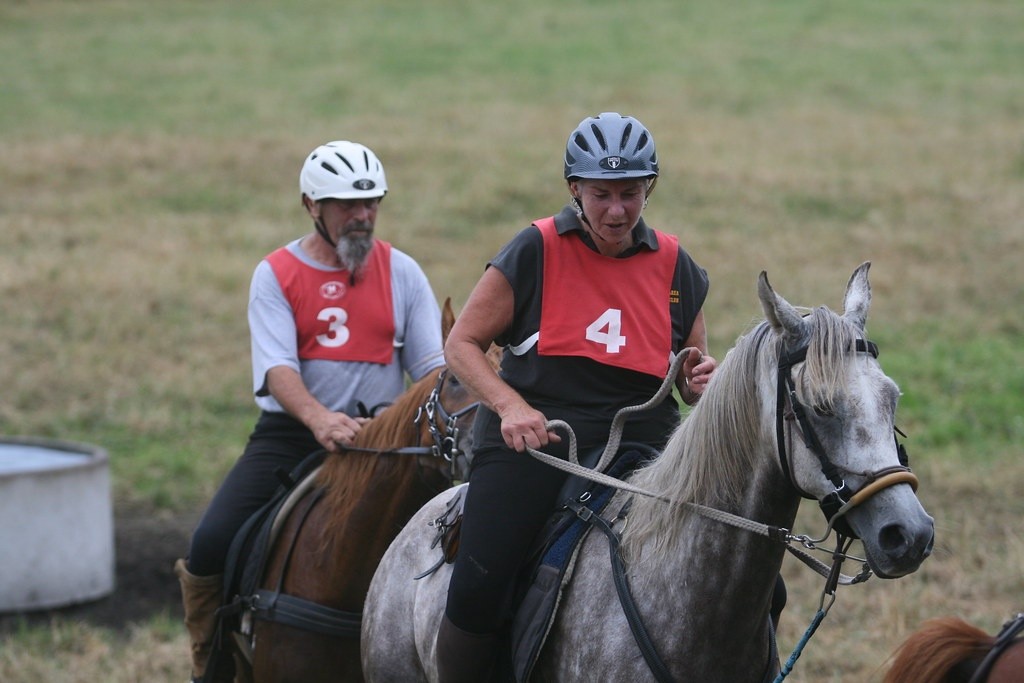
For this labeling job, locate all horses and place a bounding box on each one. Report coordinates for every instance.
[875,618,1024,683]
[228,295,503,682]
[362,261,936,683]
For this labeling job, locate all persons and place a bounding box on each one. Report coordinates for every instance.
[175,139,448,683]
[436,113,718,683]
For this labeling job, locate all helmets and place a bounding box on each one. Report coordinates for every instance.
[563,112,659,181]
[299,140,389,207]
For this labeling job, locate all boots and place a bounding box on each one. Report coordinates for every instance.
[173,559,237,683]
[435,611,496,683]
